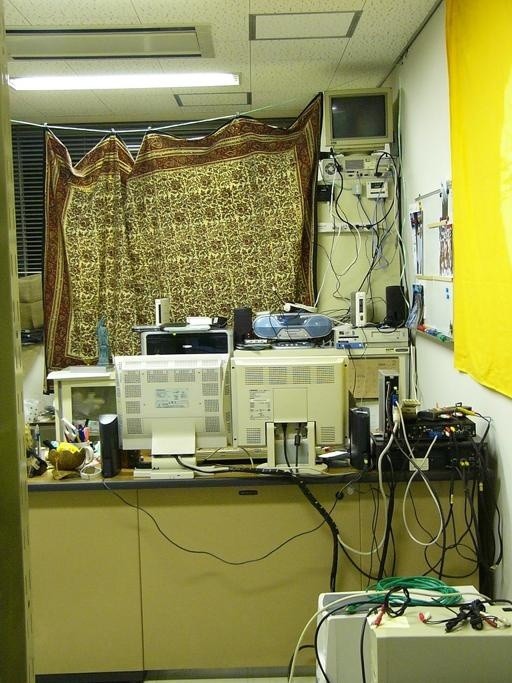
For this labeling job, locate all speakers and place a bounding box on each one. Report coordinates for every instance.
[234,306,252,346]
[99,415,116,479]
[351,405,373,471]
[385,286,404,325]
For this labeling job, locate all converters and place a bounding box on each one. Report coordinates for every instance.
[352,183,362,195]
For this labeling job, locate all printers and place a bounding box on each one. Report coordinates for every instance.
[141,317,233,355]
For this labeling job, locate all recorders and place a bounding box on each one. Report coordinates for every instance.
[253,311,333,341]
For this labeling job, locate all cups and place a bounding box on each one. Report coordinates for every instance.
[70,442,94,465]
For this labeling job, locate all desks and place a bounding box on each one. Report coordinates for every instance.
[25,445,496,677]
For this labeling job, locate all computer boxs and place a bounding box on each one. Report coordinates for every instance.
[316,584,490,683]
[368,605,511,683]
[318,157,396,180]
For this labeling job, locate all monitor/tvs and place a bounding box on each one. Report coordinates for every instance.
[232,350,349,474]
[323,87,394,151]
[114,354,231,479]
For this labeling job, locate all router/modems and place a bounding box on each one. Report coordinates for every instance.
[378,369,399,434]
[350,292,367,328]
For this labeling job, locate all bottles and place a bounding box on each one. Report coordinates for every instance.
[97,320,110,366]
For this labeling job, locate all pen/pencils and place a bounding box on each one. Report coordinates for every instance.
[61,416,91,442]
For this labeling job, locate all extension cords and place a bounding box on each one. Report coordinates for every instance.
[316,223,375,233]
